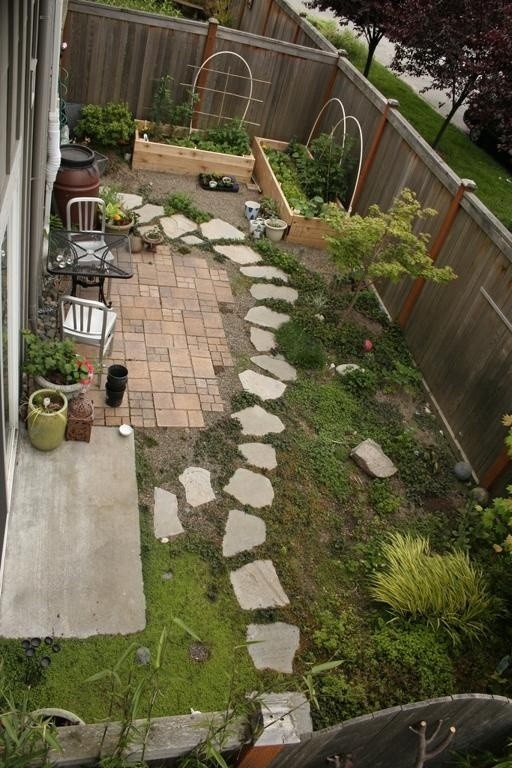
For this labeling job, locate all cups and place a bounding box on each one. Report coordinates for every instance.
[56,249,73,268]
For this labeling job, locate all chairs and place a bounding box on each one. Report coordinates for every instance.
[66,197,114,275]
[59,294,117,390]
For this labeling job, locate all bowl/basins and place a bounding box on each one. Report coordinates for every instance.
[118,424,133,437]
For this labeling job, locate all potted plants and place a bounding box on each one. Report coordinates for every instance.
[97,183,134,235]
[264,216,287,241]
[250,216,265,239]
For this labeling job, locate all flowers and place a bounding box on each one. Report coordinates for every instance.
[18,326,92,384]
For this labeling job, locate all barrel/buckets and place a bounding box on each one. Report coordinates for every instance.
[54,143,100,227]
[104,364,129,408]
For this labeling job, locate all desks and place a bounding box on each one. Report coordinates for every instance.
[46,230,134,308]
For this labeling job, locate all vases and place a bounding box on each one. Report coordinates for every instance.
[245,200,260,220]
[33,368,92,400]
